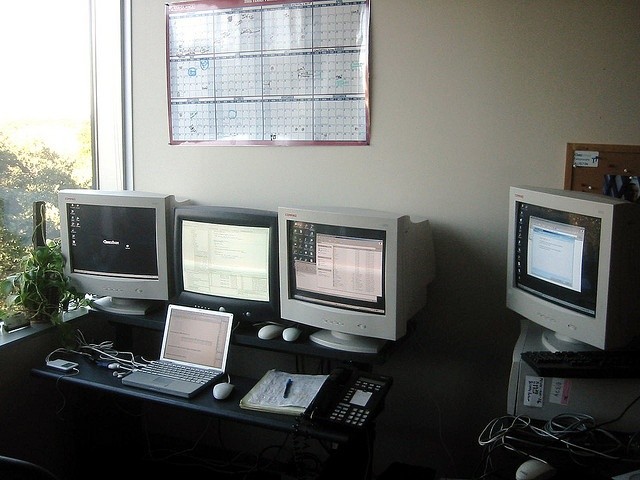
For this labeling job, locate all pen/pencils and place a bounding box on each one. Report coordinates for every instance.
[283,379,291,398]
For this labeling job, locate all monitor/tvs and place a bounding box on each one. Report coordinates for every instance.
[278,205,436,354]
[58,189,191,316]
[173,206,279,322]
[506,185,640,352]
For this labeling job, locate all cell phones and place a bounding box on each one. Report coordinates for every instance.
[46,359,79,373]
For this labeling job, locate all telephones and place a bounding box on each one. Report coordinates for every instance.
[292,366,394,469]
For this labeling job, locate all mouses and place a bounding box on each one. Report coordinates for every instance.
[515,459,557,480]
[258,325,285,340]
[283,328,302,342]
[213,382,235,400]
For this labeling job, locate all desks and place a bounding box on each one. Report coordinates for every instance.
[498,416,640,480]
[29,299,413,480]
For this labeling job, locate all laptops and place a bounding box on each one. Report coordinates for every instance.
[121,304,234,399]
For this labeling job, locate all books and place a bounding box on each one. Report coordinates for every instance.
[239,370,330,415]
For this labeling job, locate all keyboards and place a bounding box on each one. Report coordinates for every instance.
[521,350,640,378]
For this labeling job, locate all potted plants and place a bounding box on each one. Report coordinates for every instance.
[1,241,93,338]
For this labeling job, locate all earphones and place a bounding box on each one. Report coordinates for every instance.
[112,371,124,376]
[117,375,123,378]
